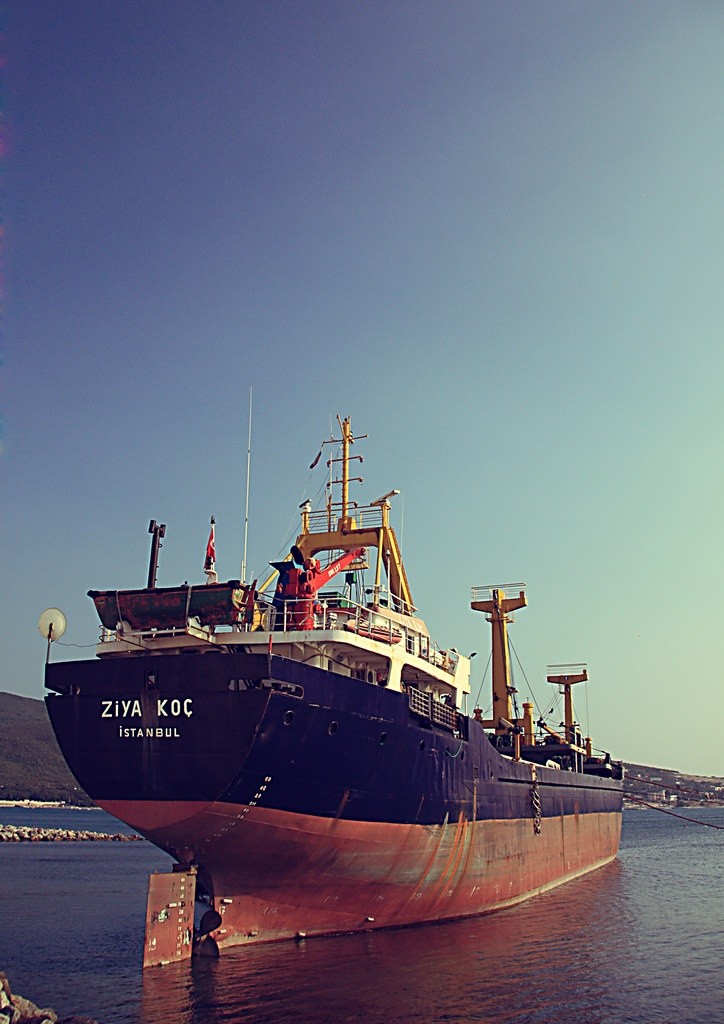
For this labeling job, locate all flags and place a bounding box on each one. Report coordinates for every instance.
[204,523,218,575]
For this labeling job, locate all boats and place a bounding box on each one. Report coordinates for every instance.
[40,406,628,968]
